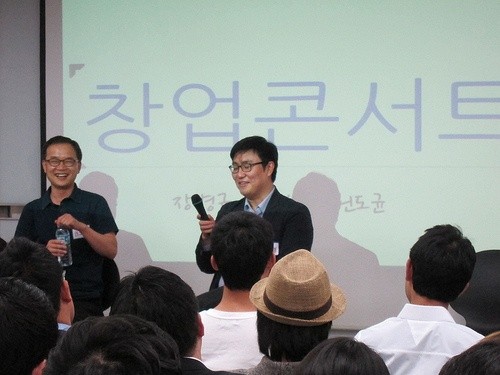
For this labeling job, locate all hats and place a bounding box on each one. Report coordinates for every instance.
[249,249,347,326]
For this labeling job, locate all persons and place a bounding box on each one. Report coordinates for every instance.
[0,276,500,375]
[195,134,314,312]
[12,135,120,328]
[0,211,499,375]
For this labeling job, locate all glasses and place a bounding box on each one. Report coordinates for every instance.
[45,158,79,167]
[229,162,264,174]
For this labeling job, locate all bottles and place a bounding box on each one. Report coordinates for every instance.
[56,227,72,266]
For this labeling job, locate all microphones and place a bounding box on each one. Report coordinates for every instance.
[191,194,210,221]
